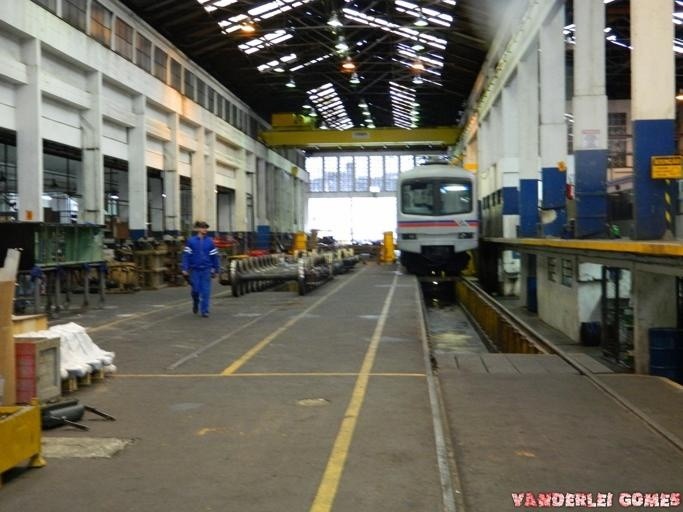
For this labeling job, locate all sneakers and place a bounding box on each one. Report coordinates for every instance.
[192,301,209,318]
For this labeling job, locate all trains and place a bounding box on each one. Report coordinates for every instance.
[396,157,480,273]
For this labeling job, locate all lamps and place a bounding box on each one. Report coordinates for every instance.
[284,79,296,88]
[326,11,361,85]
[411,76,424,85]
[413,7,428,27]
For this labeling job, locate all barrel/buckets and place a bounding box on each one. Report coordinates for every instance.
[648,327,683,385]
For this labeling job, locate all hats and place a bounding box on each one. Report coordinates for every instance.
[194,221,209,229]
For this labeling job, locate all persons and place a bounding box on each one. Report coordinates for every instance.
[181,221,221,317]
